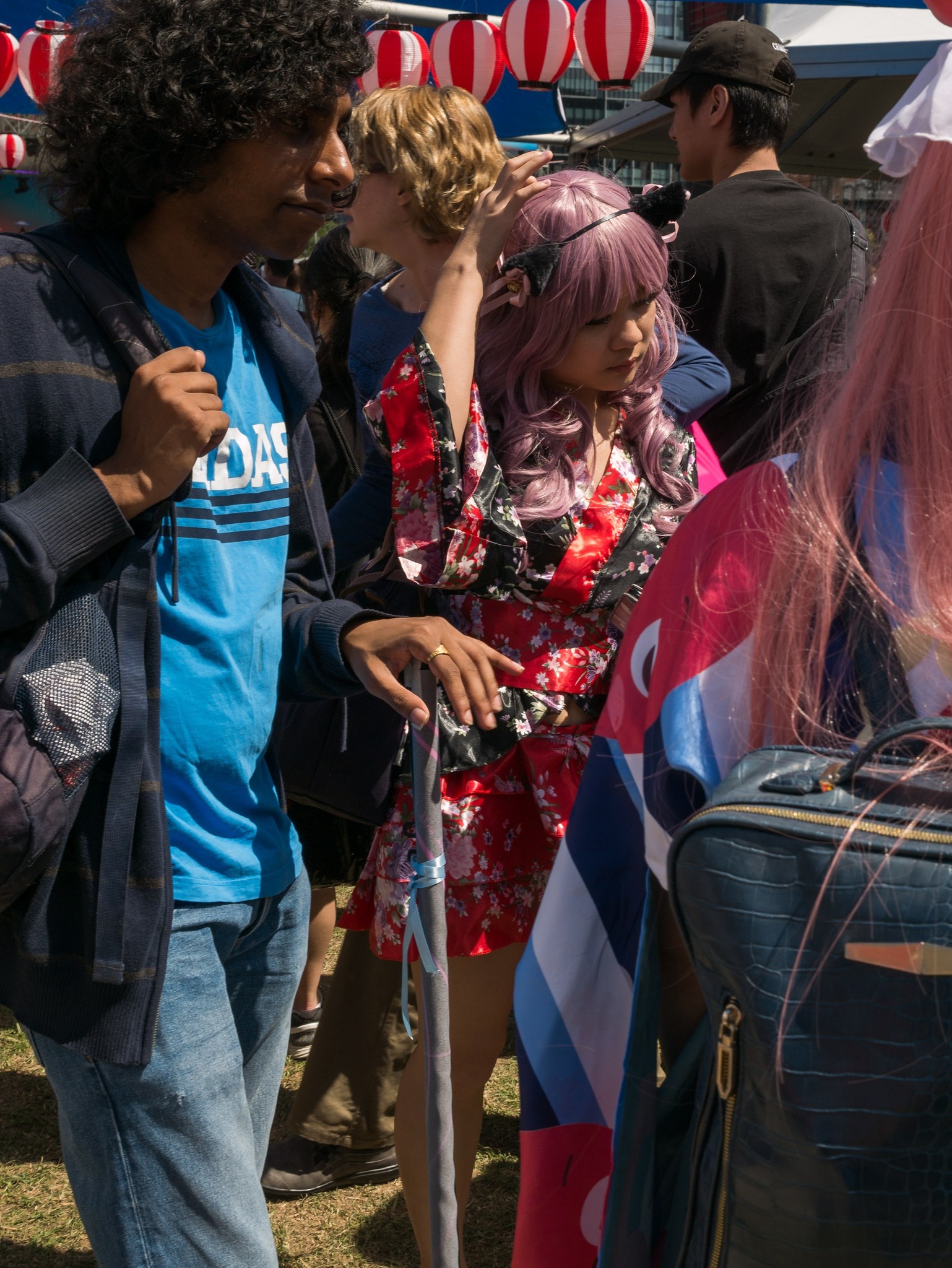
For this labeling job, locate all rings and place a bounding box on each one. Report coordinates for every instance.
[427,643,451,662]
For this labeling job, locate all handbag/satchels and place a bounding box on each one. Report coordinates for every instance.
[1,580,120,908]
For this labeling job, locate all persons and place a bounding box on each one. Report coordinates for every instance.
[366,171,695,1267]
[264,83,731,1268]
[262,223,404,1060]
[0,0,525,1268]
[640,18,874,477]
[509,42,952,1266]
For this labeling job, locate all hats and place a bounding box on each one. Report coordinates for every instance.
[639,21,796,107]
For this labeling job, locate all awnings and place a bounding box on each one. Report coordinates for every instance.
[566,0,952,179]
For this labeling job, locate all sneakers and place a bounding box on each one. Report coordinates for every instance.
[285,1003,321,1061]
[260,1136,399,1194]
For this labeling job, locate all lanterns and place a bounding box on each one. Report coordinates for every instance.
[0,0,84,169]
[355,0,654,106]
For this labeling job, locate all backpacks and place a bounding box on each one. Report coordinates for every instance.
[667,463,952,1268]
